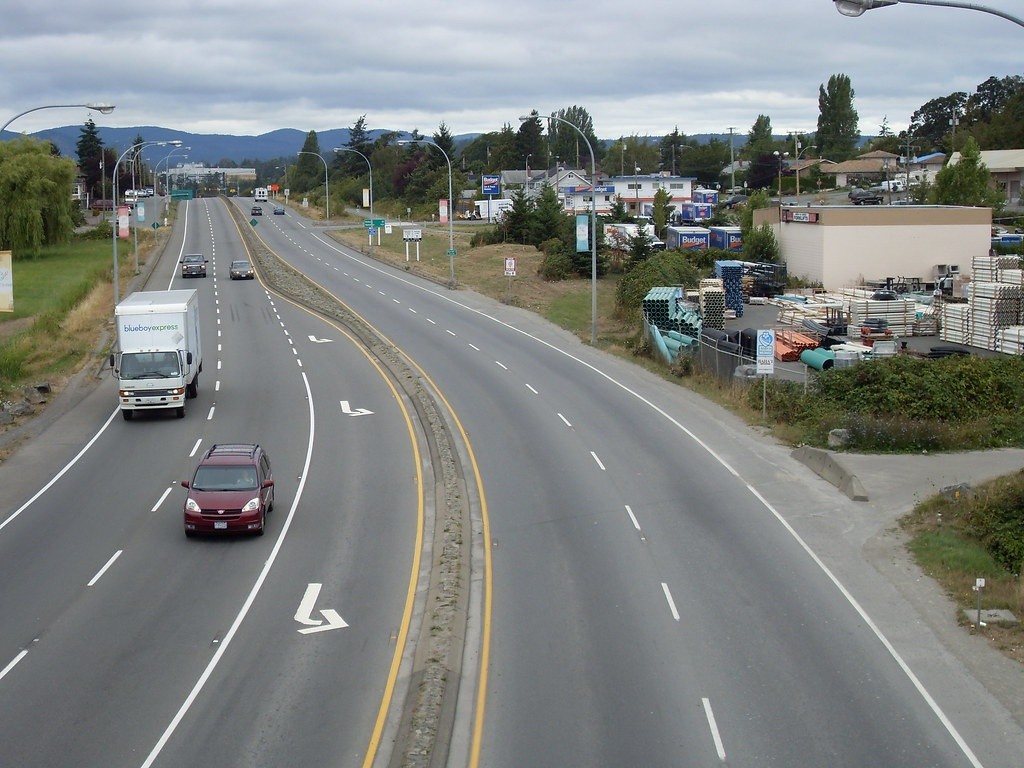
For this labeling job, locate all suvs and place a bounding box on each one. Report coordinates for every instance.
[181,445,273,540]
[180,254,208,278]
[89,200,114,211]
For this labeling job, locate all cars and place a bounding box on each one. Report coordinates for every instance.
[251,207,263,216]
[723,179,926,210]
[273,206,285,216]
[229,261,254,281]
[116,188,153,223]
[521,114,599,348]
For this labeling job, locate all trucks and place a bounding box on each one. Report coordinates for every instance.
[110,290,203,421]
[254,188,268,203]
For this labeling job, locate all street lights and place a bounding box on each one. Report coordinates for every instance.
[166,146,191,216]
[398,140,454,278]
[132,140,184,275]
[773,151,790,204]
[111,139,167,306]
[152,154,188,242]
[796,146,817,205]
[296,149,374,247]
[276,165,295,204]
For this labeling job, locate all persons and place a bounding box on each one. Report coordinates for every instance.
[237,470,253,485]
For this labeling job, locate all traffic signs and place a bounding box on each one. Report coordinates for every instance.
[365,220,386,227]
